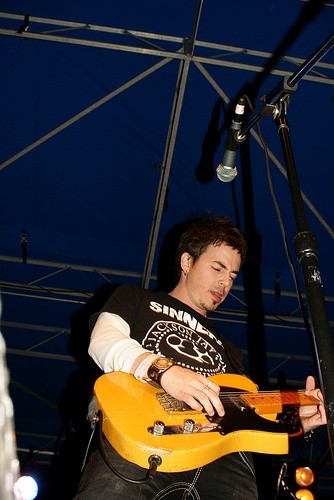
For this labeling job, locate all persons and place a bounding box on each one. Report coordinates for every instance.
[76,216,328,500]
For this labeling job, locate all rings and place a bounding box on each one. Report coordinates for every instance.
[203,386,210,394]
[206,380,213,388]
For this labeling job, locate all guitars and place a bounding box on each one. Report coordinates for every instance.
[93,372,319,472]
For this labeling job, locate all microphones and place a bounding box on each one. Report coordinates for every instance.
[217,98,245,182]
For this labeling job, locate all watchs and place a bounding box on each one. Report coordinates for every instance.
[149,356,175,383]
